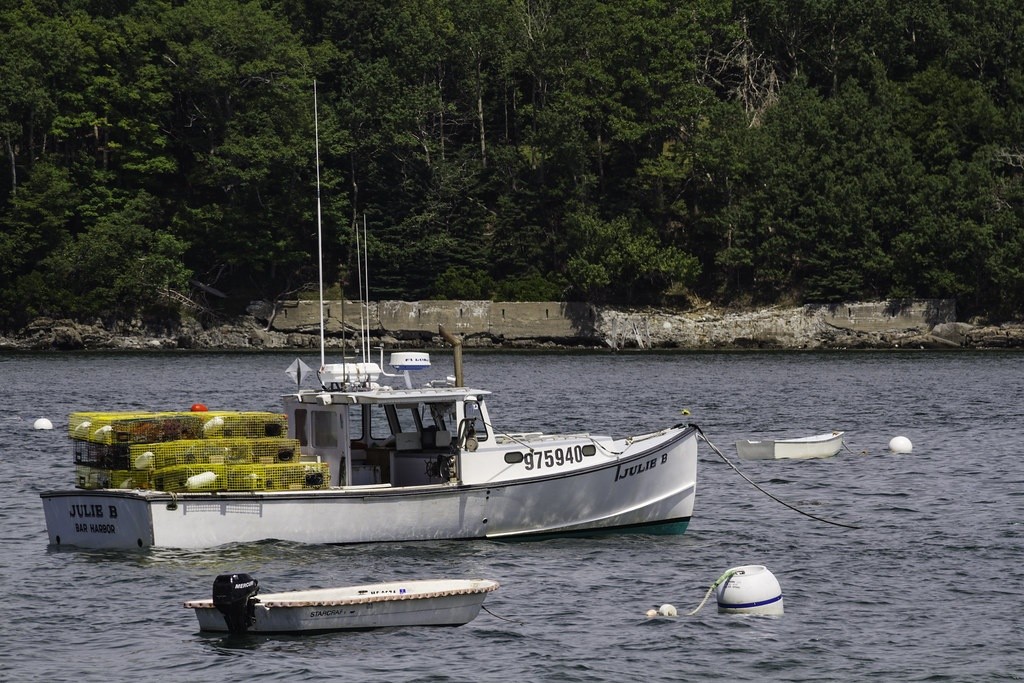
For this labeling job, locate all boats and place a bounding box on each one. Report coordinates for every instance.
[735,430,845,461]
[184,573,500,626]
[41,77,698,551]
